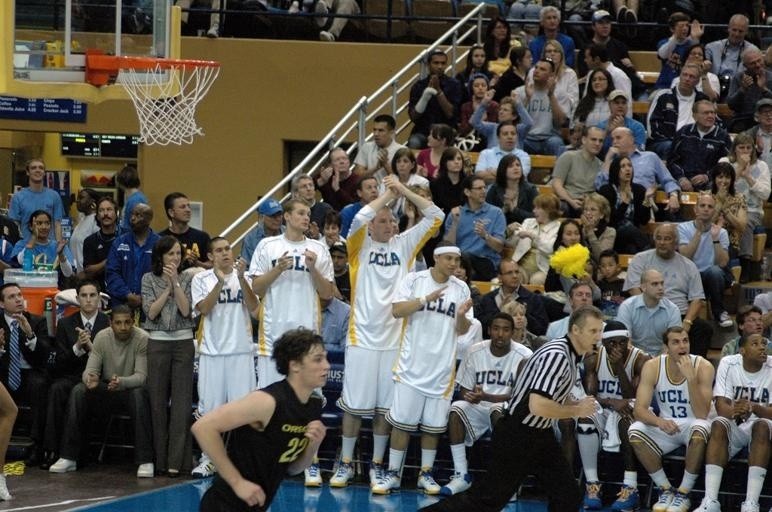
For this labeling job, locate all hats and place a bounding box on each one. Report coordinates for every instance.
[607,88,630,102]
[755,98,772,111]
[592,8,612,23]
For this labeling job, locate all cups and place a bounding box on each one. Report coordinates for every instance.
[35,263,52,277]
[45,297,65,337]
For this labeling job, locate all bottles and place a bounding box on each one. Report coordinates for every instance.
[22,244,35,271]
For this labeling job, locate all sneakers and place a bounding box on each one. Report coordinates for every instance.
[372,470,403,494]
[0,473,15,501]
[192,457,216,478]
[652,489,673,511]
[49,458,77,473]
[330,458,358,488]
[416,466,441,496]
[440,470,474,496]
[611,484,643,512]
[583,479,601,509]
[739,501,762,512]
[666,493,692,512]
[368,461,386,487]
[303,458,322,488]
[136,463,154,477]
[693,498,721,512]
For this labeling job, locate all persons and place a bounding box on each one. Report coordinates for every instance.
[1,0,772,512]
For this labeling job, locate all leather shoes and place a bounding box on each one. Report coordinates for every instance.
[168,467,181,478]
